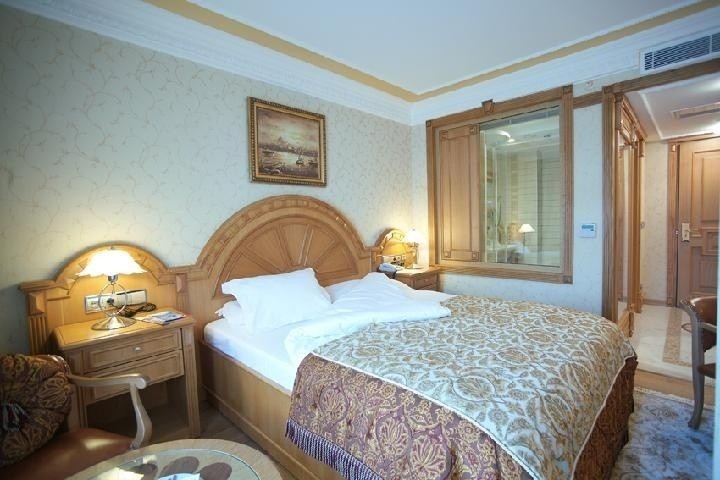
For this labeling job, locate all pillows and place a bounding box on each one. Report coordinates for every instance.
[213,266,349,331]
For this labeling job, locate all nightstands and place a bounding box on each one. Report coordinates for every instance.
[394,263,444,293]
[51,309,199,439]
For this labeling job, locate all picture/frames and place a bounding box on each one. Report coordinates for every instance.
[245,95,328,188]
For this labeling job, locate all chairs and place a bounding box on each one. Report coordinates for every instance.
[678,294,718,432]
[0,352,155,479]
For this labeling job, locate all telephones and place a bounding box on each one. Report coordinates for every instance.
[379,263,403,273]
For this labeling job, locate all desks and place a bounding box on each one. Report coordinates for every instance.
[64,437,284,480]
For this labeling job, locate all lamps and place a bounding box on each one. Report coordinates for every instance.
[402,227,422,269]
[76,244,150,331]
[517,220,536,253]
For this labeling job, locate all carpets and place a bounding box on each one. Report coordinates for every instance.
[610,383,716,479]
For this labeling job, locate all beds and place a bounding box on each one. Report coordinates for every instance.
[196,193,640,480]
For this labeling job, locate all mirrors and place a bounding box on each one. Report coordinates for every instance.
[424,79,577,285]
